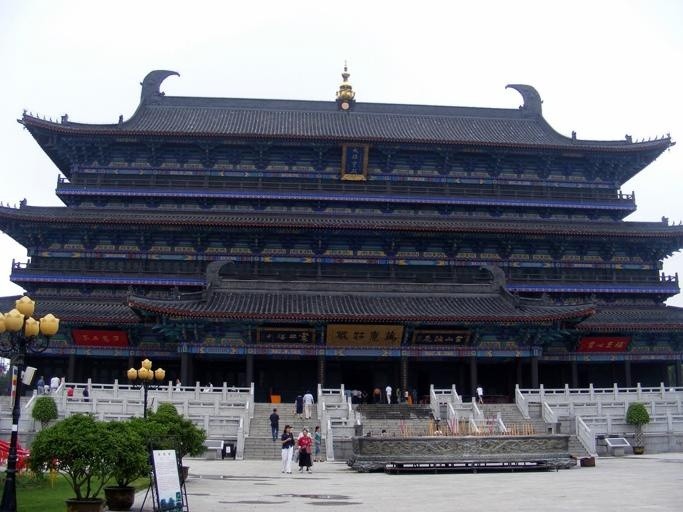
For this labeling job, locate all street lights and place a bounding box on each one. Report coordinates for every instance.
[126,359,166,420]
[1,296,59,511]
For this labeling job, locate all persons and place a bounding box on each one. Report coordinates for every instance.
[476,383,485,405]
[344,382,418,406]
[433,426,443,435]
[50,374,60,392]
[380,429,386,436]
[82,385,90,402]
[66,384,74,401]
[37,375,45,396]
[268,389,323,475]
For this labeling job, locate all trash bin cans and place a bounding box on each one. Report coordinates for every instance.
[221,443,236,460]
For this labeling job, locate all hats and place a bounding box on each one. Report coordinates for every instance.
[285,425,292,428]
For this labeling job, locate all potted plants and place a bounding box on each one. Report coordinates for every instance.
[626,403,650,454]
[97,418,152,512]
[132,403,208,487]
[24,414,125,512]
